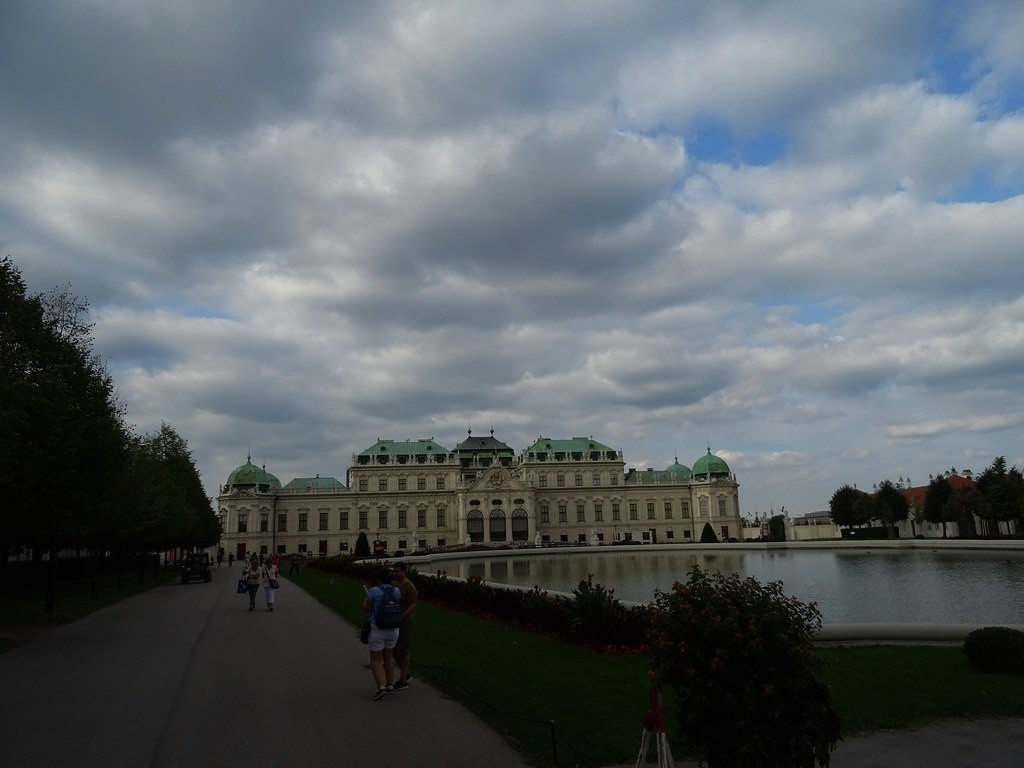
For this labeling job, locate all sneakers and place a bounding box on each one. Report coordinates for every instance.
[371,688,394,700]
[406,674,412,683]
[394,680,408,690]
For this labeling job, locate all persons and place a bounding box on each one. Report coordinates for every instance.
[229,552,234,566]
[217,553,222,567]
[510,540,528,548]
[363,561,418,701]
[261,558,278,612]
[242,560,262,611]
[424,544,449,552]
[301,549,312,556]
[578,539,580,546]
[350,547,352,555]
[244,551,302,578]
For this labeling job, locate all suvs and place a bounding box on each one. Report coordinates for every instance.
[180,553,212,584]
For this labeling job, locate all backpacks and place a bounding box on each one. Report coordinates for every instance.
[372,585,400,630]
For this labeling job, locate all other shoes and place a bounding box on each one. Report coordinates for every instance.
[297,574,299,576]
[289,574,292,577]
[248,606,254,611]
[266,607,273,611]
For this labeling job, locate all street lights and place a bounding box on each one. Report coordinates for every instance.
[272,494,277,554]
[688,484,695,543]
[376,533,379,540]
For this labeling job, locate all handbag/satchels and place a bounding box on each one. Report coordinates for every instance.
[237,580,247,593]
[270,578,280,589]
